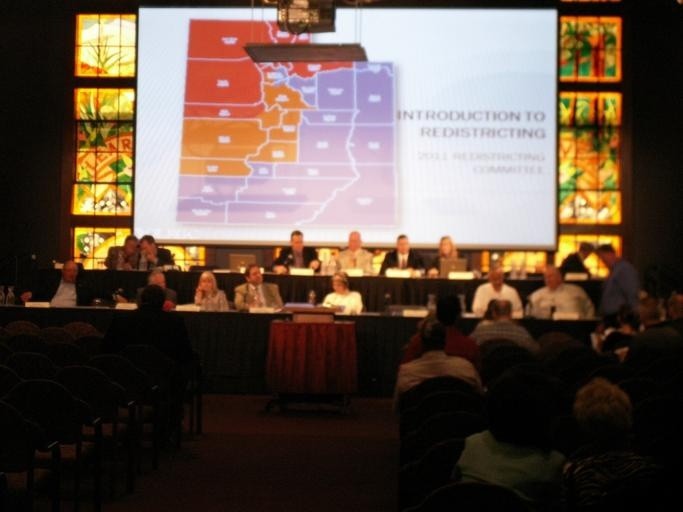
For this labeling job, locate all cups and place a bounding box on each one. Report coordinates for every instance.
[96,261,103,269]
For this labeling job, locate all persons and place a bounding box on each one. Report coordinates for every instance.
[20,260,103,306]
[427,236,472,277]
[332,232,374,275]
[389,294,682,512]
[525,266,593,317]
[102,285,193,448]
[193,270,230,312]
[103,235,140,271]
[234,263,284,313]
[595,242,642,331]
[560,242,595,279]
[320,270,362,313]
[140,236,175,272]
[271,231,321,274]
[378,234,424,277]
[472,259,522,311]
[135,273,178,309]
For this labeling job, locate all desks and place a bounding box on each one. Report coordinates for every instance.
[0,263,606,400]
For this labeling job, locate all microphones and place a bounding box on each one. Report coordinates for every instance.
[549,306,557,321]
[385,293,391,306]
[112,288,123,296]
[282,254,293,265]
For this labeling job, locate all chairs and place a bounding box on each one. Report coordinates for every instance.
[0,319,203,512]
[393,322,683,512]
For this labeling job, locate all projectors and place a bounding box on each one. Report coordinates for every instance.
[276,0,336,33]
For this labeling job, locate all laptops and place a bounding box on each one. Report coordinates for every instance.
[439,257,468,279]
[228,253,257,274]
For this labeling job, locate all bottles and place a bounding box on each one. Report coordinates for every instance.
[524,300,535,317]
[508,258,527,280]
[138,251,146,270]
[5,286,14,304]
[305,289,315,305]
[239,262,245,274]
[383,295,392,316]
[328,253,340,274]
[115,249,124,269]
